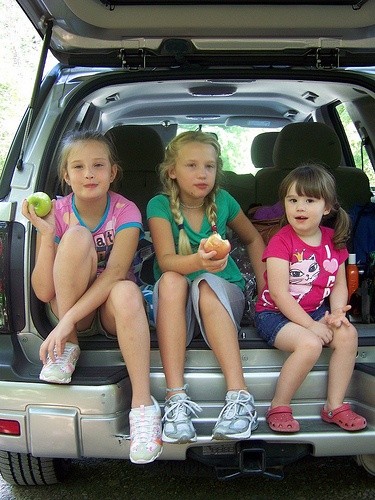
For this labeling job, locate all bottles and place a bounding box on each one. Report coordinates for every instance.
[345,253,359,304]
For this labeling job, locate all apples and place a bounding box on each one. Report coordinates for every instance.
[204,234,231,259]
[26,191,51,217]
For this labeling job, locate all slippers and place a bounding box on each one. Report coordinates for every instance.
[321,403,366,430]
[266,406,300,432]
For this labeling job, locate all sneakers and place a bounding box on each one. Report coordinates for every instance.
[212,390,259,439]
[129,395,163,464]
[39,341,80,383]
[162,393,202,443]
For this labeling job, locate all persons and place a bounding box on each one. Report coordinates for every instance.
[255,166,367,432]
[22,130,164,464]
[147,131,267,444]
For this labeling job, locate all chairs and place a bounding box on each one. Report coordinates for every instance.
[250,133,280,169]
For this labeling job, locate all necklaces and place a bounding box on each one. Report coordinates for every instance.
[180,201,205,208]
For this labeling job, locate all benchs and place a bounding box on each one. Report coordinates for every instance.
[101,122,371,233]
